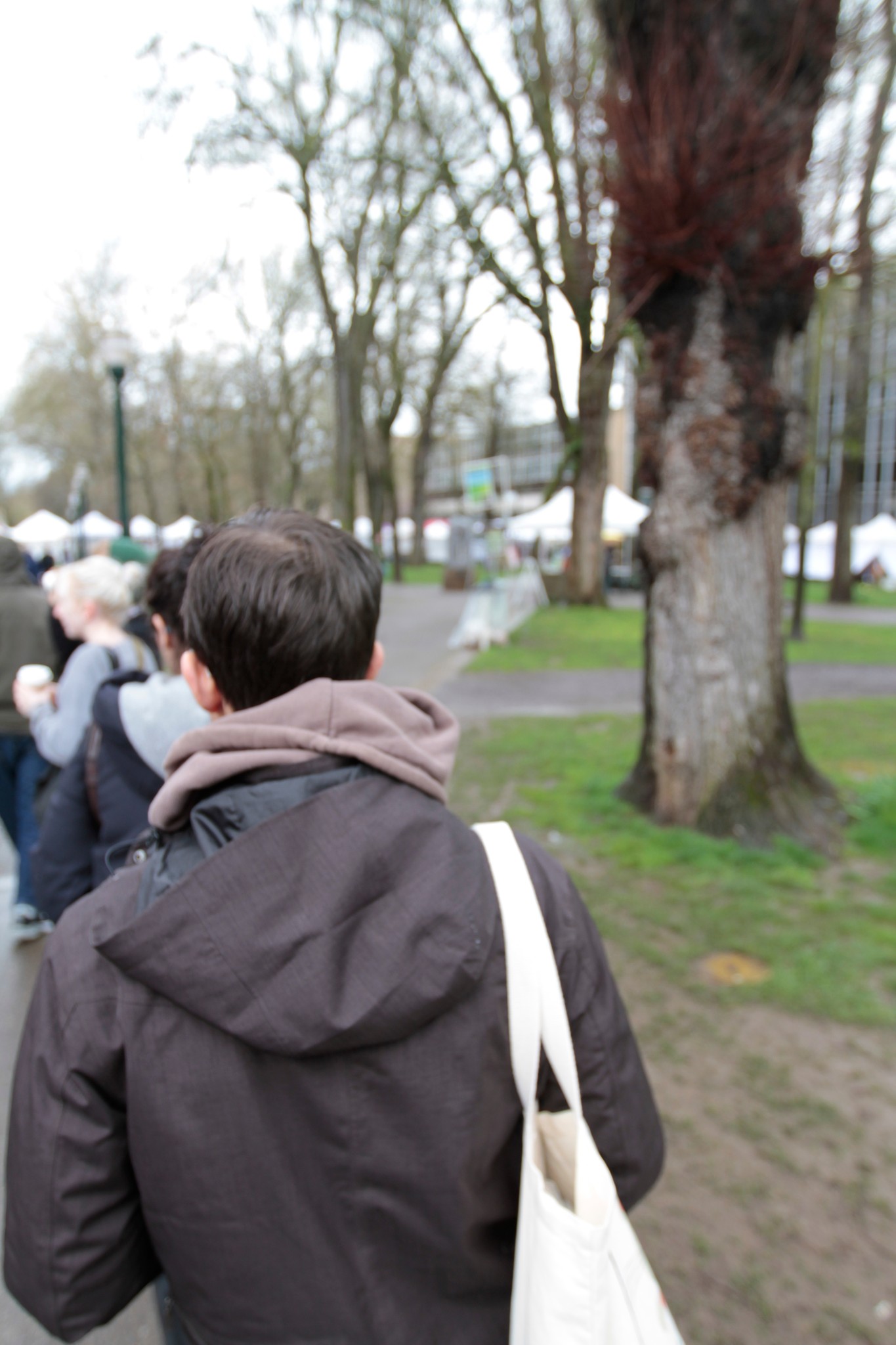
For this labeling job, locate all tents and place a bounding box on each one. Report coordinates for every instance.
[0,468,896,602]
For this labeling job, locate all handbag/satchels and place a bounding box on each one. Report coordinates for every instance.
[470,815,686,1345]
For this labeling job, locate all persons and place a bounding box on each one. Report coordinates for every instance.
[1,506,665,1345]
[29,531,231,934]
[0,526,160,943]
